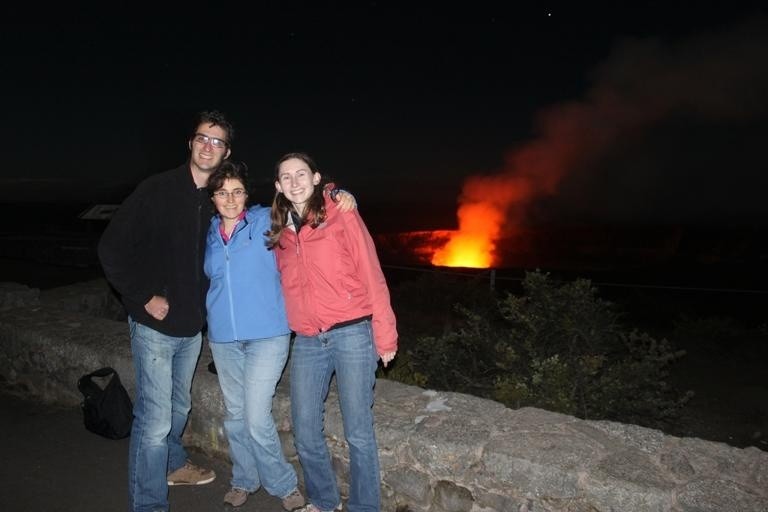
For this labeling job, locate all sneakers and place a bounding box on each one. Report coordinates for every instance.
[164,459,216,486]
[280,488,344,512]
[225,484,261,507]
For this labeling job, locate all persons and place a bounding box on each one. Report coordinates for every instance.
[262,153,399,512]
[203,161,359,511]
[98,111,234,512]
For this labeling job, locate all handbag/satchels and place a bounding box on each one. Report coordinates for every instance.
[77,368,133,439]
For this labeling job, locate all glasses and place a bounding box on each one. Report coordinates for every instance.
[192,133,230,149]
[213,189,247,199]
